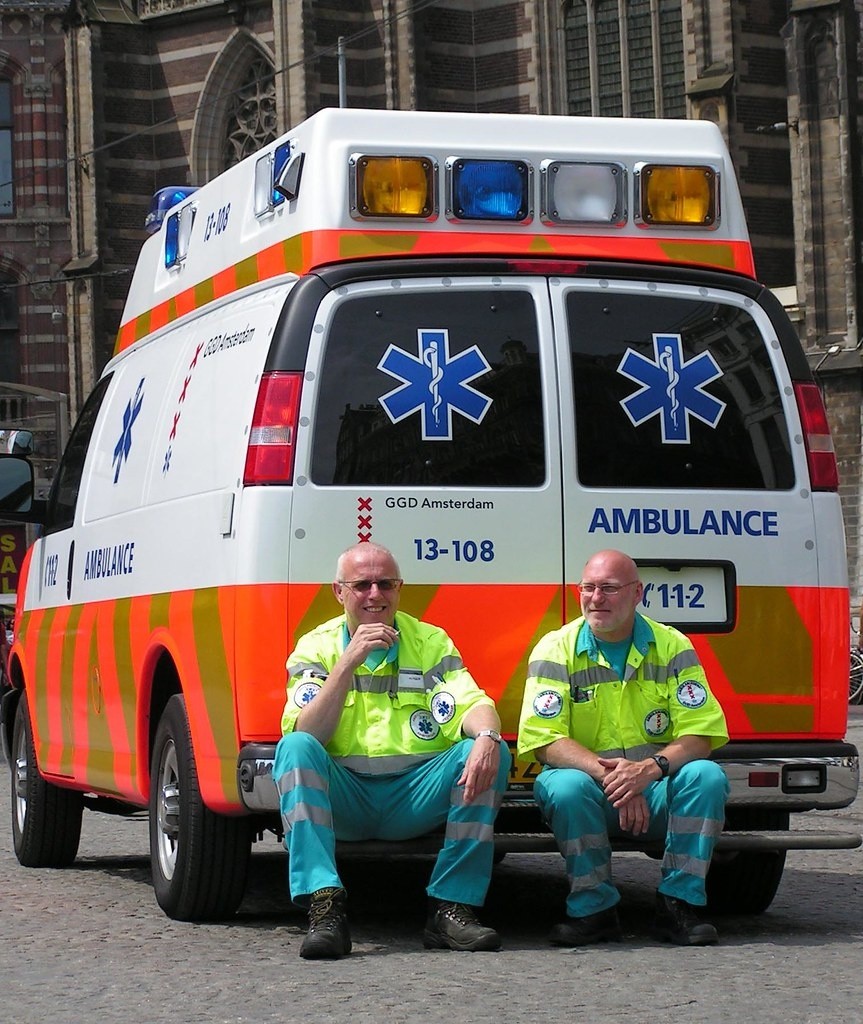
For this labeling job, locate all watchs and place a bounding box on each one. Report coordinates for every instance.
[477,730,501,744]
[650,756,669,778]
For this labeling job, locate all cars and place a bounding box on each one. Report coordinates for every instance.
[0,592,20,643]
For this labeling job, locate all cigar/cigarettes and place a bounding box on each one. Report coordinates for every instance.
[394,630,401,635]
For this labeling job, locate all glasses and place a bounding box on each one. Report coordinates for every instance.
[576,580,638,595]
[339,579,402,592]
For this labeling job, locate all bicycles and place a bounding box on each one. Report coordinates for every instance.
[845,647,863,703]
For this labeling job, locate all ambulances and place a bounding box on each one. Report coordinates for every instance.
[1,100,861,922]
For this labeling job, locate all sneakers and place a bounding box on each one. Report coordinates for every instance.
[300,887,352,958]
[549,906,620,945]
[423,896,501,951]
[653,887,718,946]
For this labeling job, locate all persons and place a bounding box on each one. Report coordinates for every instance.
[0,612,14,686]
[274,542,512,956]
[517,549,731,944]
